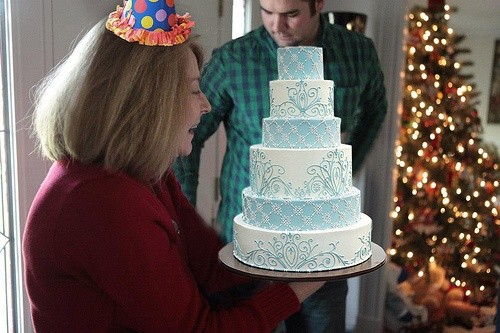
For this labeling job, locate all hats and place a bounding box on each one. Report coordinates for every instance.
[106,0,195,44]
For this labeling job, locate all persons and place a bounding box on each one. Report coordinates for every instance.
[171,0,388,333]
[20,14,327,333]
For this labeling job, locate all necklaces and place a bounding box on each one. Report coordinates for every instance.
[149,182,180,235]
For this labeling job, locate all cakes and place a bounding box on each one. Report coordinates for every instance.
[232,47,374,273]
